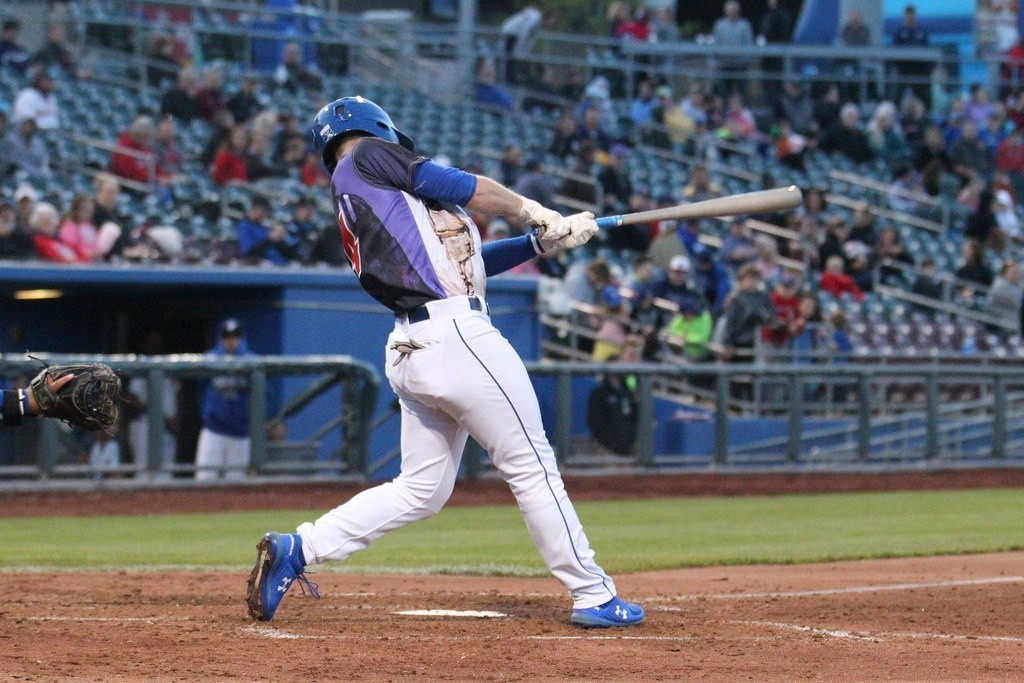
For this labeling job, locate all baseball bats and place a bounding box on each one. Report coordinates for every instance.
[529,184,804,239]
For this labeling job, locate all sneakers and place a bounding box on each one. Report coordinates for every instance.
[570,596,645,626]
[245,532,320,622]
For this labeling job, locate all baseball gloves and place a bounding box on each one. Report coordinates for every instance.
[32,360,124,434]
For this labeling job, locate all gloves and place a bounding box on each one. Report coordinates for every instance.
[508,196,571,240]
[542,211,599,256]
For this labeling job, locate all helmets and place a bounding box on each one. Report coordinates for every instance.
[223,319,240,334]
[311,96,413,177]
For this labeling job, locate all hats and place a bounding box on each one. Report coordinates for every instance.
[669,255,690,272]
[610,144,629,160]
[15,186,35,203]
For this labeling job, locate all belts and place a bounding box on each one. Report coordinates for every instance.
[407,296,481,325]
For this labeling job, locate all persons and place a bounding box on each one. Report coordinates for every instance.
[275,41,322,92]
[587,340,639,454]
[161,60,330,189]
[0,19,26,70]
[149,35,180,87]
[36,22,71,66]
[158,119,181,166]
[503,7,557,90]
[0,354,121,440]
[538,67,555,95]
[1,172,346,267]
[551,0,1024,402]
[469,138,561,278]
[193,317,253,481]
[110,116,182,186]
[472,56,515,108]
[83,372,187,480]
[245,96,645,627]
[13,66,60,130]
